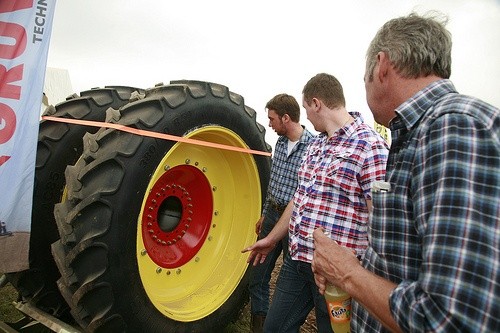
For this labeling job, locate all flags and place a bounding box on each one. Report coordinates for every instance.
[0,0,57,276]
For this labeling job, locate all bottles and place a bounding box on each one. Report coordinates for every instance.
[324,277,353,333]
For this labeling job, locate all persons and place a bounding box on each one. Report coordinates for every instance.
[309,10,500,333]
[240,70,394,333]
[245,93,319,333]
